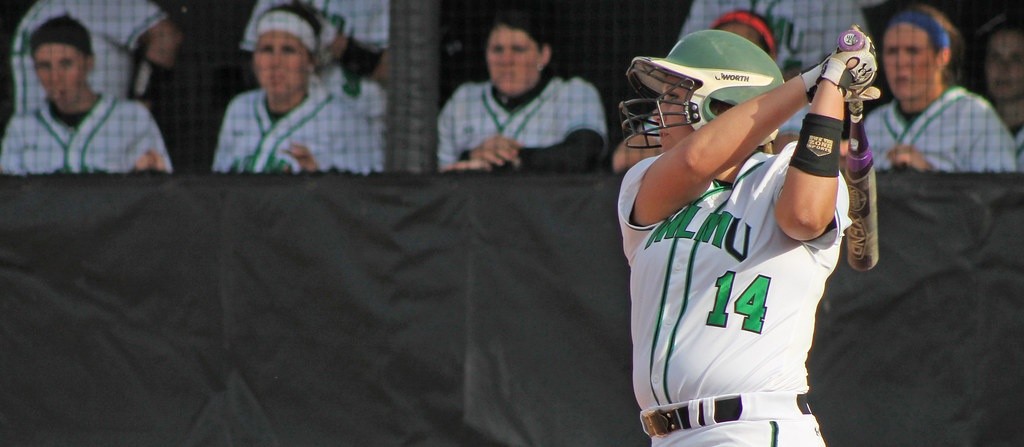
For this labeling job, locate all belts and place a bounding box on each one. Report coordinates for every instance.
[642,393,815,438]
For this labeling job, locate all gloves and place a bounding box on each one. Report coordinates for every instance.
[799,23,881,104]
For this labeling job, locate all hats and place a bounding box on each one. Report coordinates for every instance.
[31,13,92,56]
[256,2,320,52]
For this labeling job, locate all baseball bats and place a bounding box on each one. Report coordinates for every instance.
[837,29,879,270]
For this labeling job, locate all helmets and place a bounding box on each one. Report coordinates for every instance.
[618,30,784,145]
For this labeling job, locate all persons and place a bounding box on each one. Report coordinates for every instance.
[617,24,881,447]
[0,0,182,174]
[436,12,609,171]
[612,1,1023,173]
[211,0,390,176]
